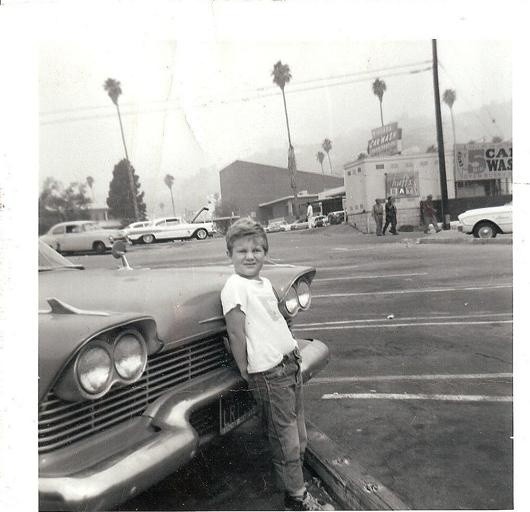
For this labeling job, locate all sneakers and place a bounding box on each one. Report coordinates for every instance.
[285,488,335,510]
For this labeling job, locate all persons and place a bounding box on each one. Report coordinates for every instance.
[221,217,335,510]
[306,201,316,229]
[383,197,399,235]
[374,198,384,237]
[421,194,442,235]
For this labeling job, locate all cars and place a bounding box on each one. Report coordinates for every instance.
[39,240,329,511]
[457,201,514,238]
[266,211,345,233]
[122,217,215,244]
[39,220,132,255]
